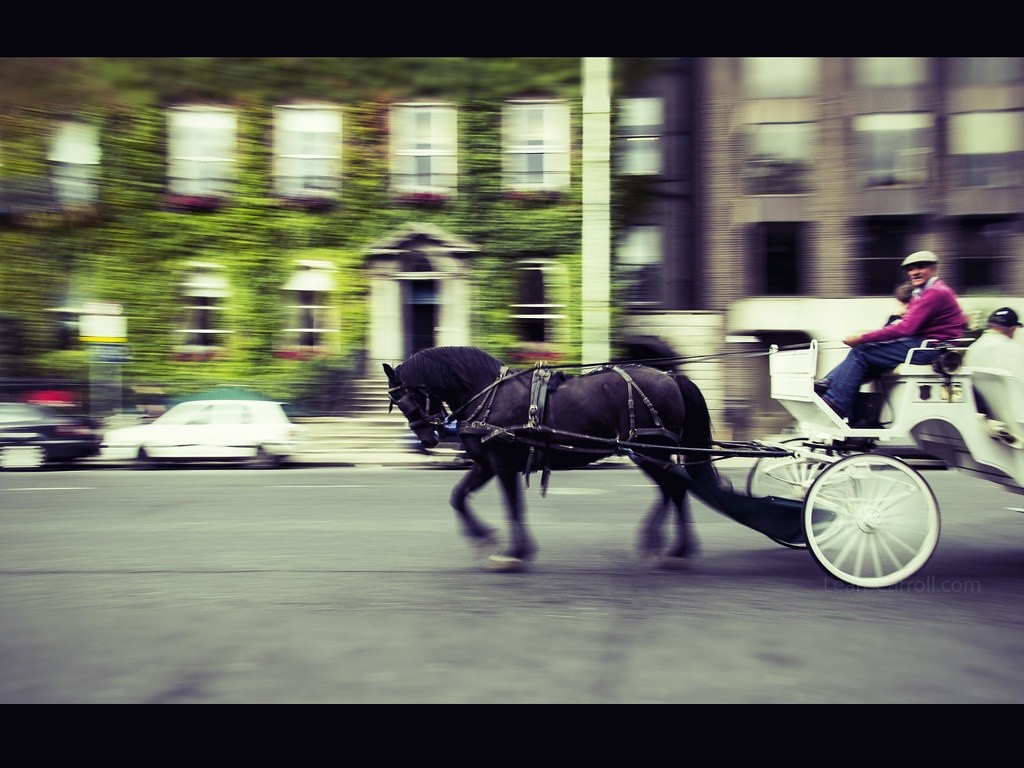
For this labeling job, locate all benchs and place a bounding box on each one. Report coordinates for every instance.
[859,337,979,428]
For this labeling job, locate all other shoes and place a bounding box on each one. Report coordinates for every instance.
[814,381,827,397]
[815,390,850,426]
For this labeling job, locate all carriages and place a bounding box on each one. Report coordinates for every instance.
[381,312,1024,588]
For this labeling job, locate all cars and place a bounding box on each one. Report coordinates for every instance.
[98,401,292,467]
[0,403,103,471]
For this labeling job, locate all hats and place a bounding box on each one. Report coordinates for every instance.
[901,251,938,266]
[988,307,1022,327]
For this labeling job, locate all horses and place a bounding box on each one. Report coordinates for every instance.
[382,346,725,572]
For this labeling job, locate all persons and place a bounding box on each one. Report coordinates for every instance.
[962,307,1024,440]
[814,251,968,420]
